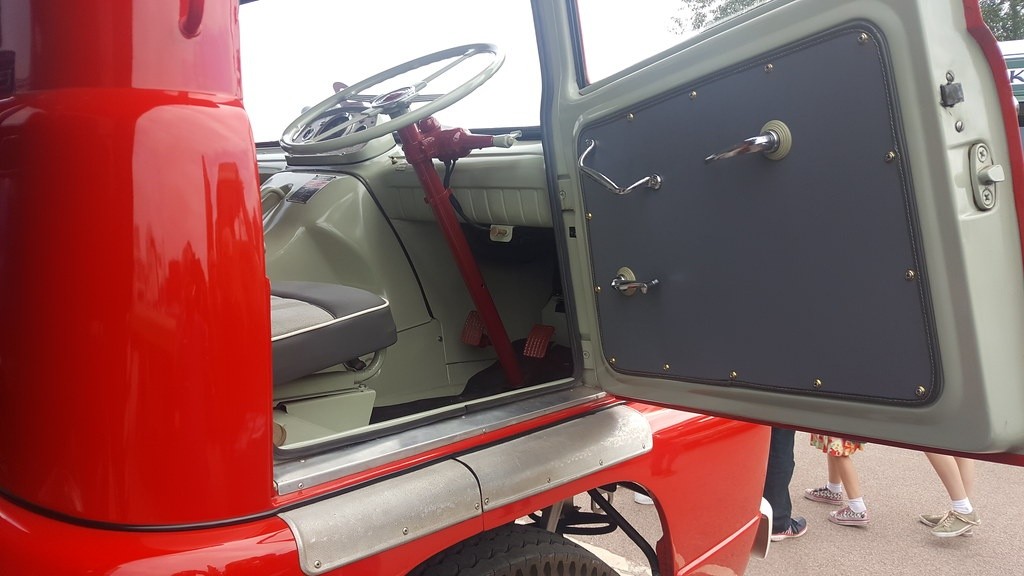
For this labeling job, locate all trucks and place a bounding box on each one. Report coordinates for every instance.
[1,0,1024,576]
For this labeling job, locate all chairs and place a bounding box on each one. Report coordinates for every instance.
[268,280,397,404]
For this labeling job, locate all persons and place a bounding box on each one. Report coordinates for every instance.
[804,433,870,527]
[763,426,807,541]
[919,451,981,538]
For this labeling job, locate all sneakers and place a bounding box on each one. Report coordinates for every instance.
[771,516,808,542]
[803,485,843,505]
[828,506,870,525]
[634,492,654,505]
[918,509,981,538]
